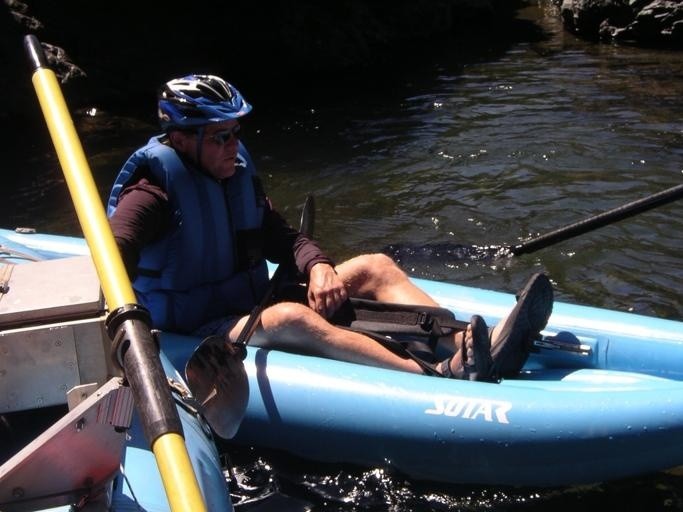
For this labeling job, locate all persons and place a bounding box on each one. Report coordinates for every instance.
[107,75,553,381]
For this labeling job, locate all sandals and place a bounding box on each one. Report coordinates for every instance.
[423,272,553,380]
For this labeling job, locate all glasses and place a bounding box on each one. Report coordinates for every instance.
[214,125,240,146]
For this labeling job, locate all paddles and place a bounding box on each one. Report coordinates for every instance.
[184,187,314,439]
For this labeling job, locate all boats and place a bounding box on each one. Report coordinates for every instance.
[2,230,682,487]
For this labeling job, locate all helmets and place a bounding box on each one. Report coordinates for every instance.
[158,74,253,128]
